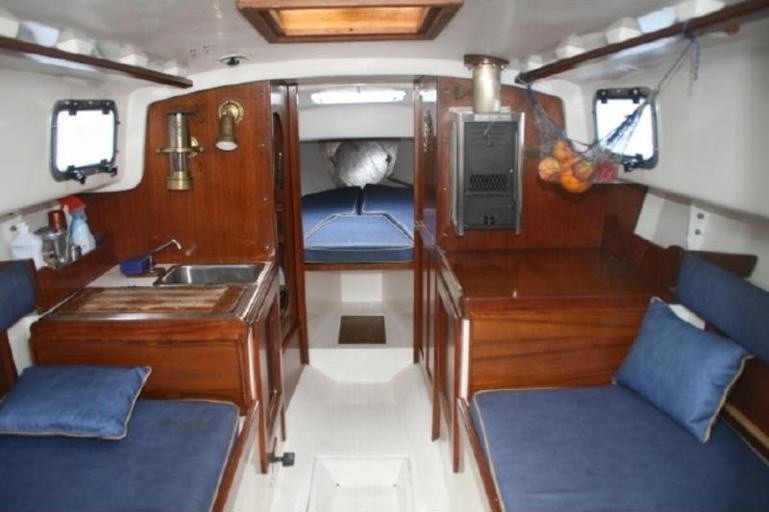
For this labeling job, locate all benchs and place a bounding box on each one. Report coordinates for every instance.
[458,246,768,512]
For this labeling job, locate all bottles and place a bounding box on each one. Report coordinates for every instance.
[34,224,68,268]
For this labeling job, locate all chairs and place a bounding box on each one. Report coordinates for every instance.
[1,259,260,511]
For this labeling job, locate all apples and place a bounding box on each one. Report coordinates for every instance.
[537,139,617,194]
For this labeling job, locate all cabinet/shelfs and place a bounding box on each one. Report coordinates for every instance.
[28,267,304,475]
[74,80,310,413]
[433,249,673,474]
[413,75,649,403]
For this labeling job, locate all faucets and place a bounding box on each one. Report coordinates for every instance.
[146,238,182,270]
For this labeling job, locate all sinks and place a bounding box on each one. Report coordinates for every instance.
[153,261,272,285]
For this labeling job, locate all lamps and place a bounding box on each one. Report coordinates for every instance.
[156,104,204,192]
[215,99,244,152]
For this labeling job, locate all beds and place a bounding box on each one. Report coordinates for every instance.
[299,183,414,350]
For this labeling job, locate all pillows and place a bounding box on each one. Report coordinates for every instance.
[1,363,153,444]
[611,293,755,446]
